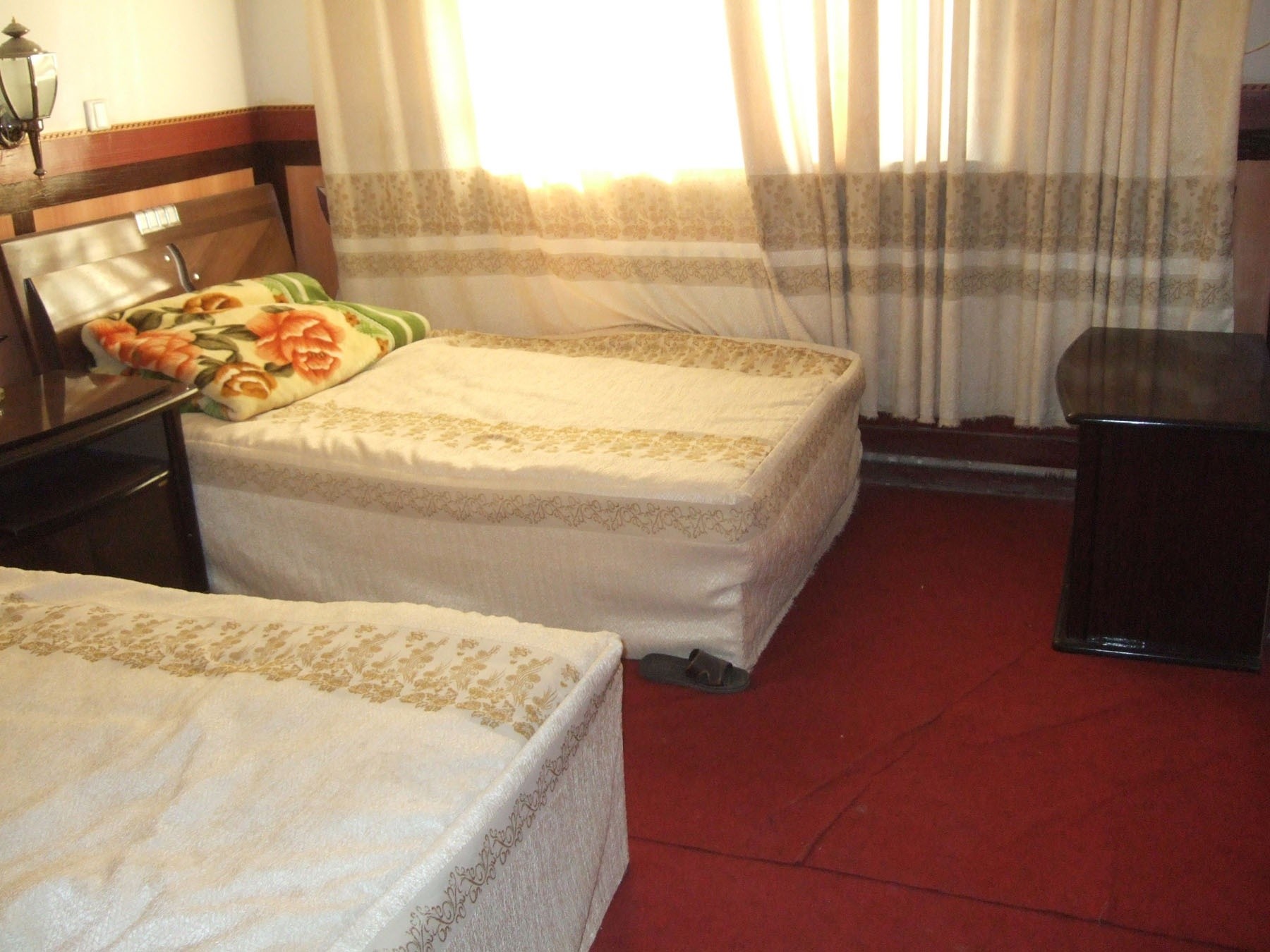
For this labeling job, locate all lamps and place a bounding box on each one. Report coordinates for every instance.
[0,17,60,182]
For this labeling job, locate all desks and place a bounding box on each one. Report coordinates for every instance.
[1047,323,1269,675]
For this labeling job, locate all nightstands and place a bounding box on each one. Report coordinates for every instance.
[0,368,208,593]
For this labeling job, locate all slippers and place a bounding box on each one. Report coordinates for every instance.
[640,648,750,693]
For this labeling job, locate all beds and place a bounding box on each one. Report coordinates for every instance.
[0,566,634,952]
[0,181,867,678]
